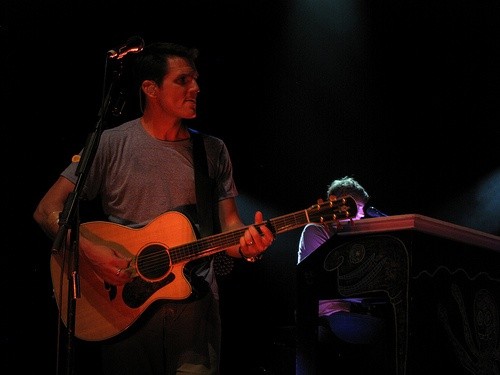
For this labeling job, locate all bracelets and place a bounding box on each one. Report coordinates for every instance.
[238,245,265,264]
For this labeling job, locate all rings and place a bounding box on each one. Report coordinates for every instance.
[116,269,120,276]
[247,241,254,246]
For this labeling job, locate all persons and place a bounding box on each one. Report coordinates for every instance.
[33,42,274,375]
[297,176,383,344]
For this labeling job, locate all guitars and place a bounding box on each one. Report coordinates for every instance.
[49,191,360,342]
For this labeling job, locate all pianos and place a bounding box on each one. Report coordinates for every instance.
[325,214,500,252]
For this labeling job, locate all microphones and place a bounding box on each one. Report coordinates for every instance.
[108,37,144,58]
[367,207,387,217]
